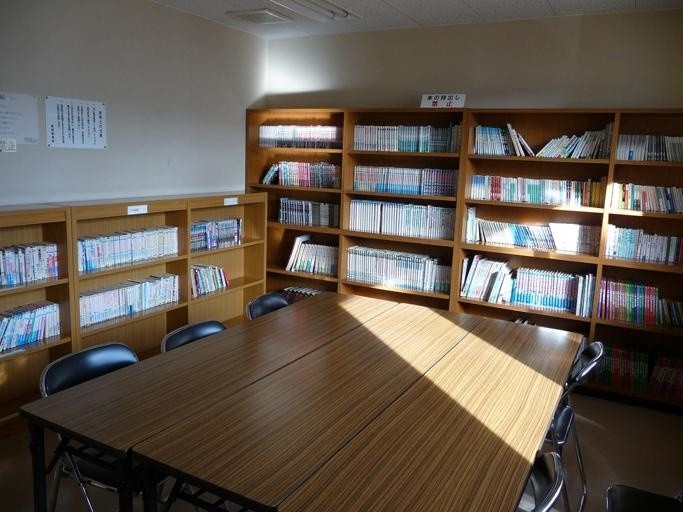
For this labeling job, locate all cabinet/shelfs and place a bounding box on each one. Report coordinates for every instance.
[177,191,267,330]
[0,201,74,434]
[453,106,673,412]
[48,194,189,366]
[245,107,466,314]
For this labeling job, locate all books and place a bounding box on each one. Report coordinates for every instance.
[460,207,601,318]
[282,287,321,304]
[278,198,338,275]
[352,124,462,196]
[0,213,245,355]
[347,199,457,292]
[258,124,341,189]
[469,122,613,208]
[610,132,683,215]
[596,223,682,328]
[591,334,683,407]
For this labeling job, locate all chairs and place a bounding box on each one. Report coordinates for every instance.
[159,295,290,352]
[39,342,195,512]
[514,339,604,512]
[605,483,683,511]
